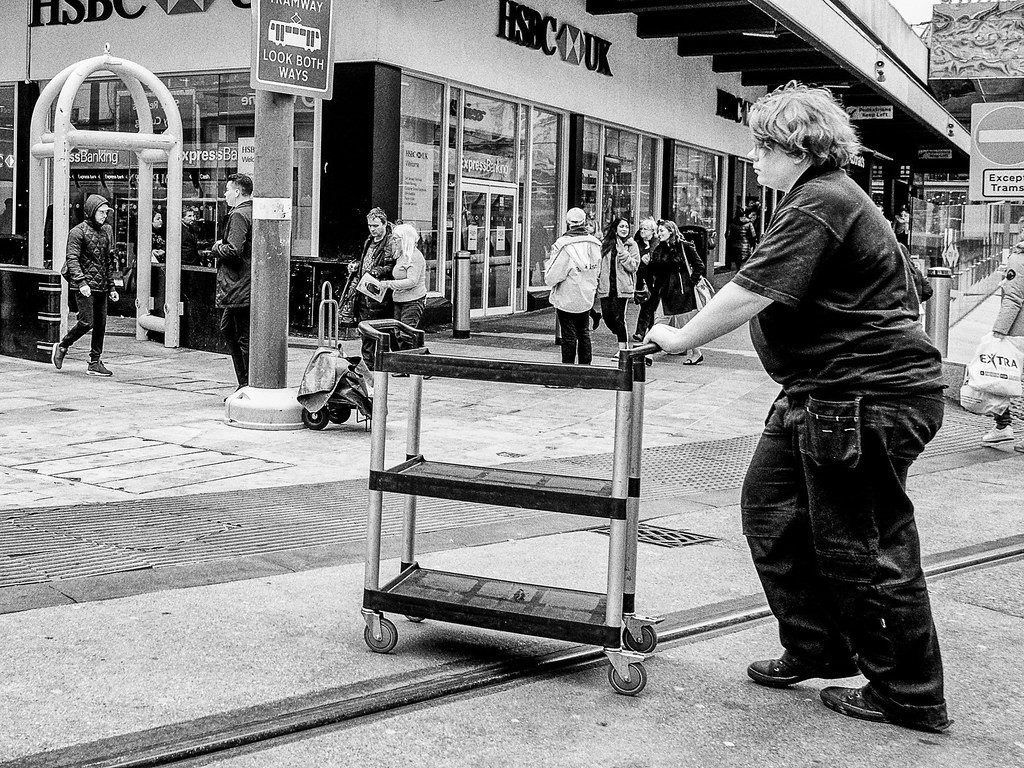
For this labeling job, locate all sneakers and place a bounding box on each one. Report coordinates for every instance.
[86,359,112,376]
[820,686,941,731]
[982,424,1014,442]
[1015,440,1024,452]
[51,342,68,369]
[748,651,861,687]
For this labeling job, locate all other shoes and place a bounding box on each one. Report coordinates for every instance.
[392,370,410,377]
[632,333,643,341]
[223,382,248,403]
[610,351,620,361]
[423,374,431,379]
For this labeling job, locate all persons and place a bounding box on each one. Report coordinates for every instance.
[983,216,1024,452]
[645,79,957,734]
[480,216,550,286]
[545,206,603,390]
[348,204,433,379]
[723,206,757,272]
[876,201,909,248]
[39,193,199,377]
[212,174,253,403]
[582,217,703,365]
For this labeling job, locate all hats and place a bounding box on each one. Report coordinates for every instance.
[567,207,586,226]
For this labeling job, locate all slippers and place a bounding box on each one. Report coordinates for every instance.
[683,353,703,365]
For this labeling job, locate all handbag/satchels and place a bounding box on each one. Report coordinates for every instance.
[635,283,651,305]
[61,259,70,283]
[339,275,360,324]
[694,274,716,311]
[968,333,1022,397]
[960,364,1011,416]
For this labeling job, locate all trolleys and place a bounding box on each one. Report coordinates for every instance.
[360,320,663,694]
[303,281,388,431]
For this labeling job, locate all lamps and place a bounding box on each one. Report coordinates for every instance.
[876,69,886,82]
[948,129,954,137]
[823,82,854,88]
[742,30,780,38]
[874,44,886,70]
[948,114,954,128]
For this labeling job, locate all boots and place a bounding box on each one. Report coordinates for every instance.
[590,309,601,330]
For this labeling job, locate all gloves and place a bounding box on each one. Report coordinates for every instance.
[691,271,700,282]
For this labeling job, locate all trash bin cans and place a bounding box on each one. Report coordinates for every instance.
[925,268,952,358]
[678,224,714,286]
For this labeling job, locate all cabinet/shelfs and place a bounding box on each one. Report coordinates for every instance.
[582,149,621,241]
[621,171,632,225]
